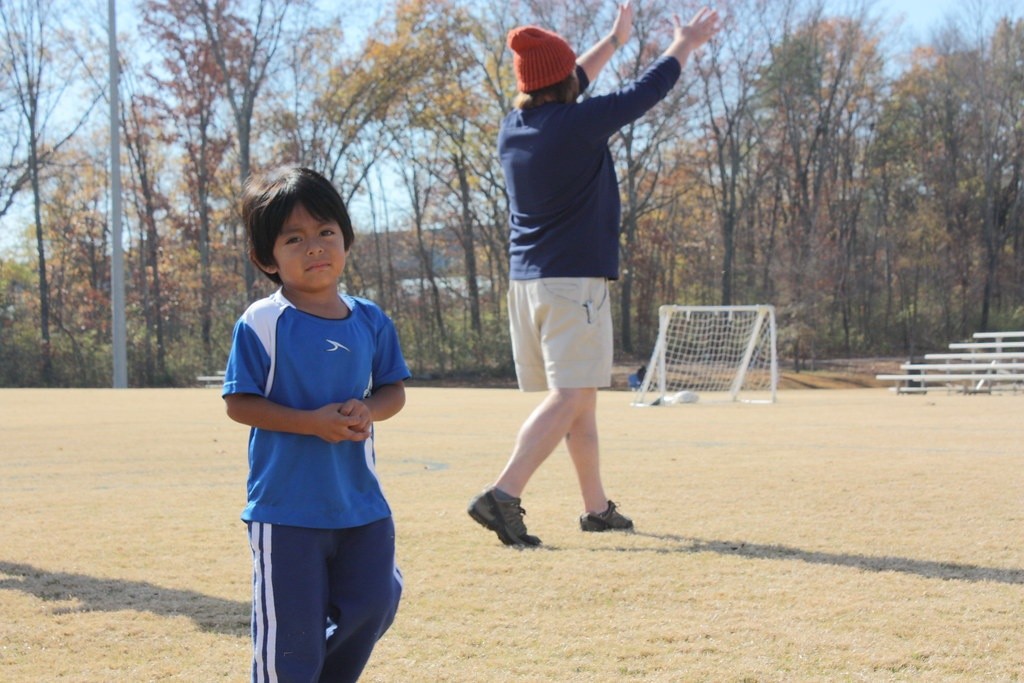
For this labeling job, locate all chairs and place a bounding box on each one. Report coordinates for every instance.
[629,373,642,392]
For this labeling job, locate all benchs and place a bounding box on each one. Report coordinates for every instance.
[875,331,1024,395]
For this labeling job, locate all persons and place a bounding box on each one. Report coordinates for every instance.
[468,0,721,549]
[222,167,412,683]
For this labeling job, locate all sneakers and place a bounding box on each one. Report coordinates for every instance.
[580,499,635,532]
[468,487,542,548]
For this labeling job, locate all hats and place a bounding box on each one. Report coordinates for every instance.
[506,26,577,93]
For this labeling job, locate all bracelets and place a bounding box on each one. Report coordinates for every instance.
[609,35,619,49]
